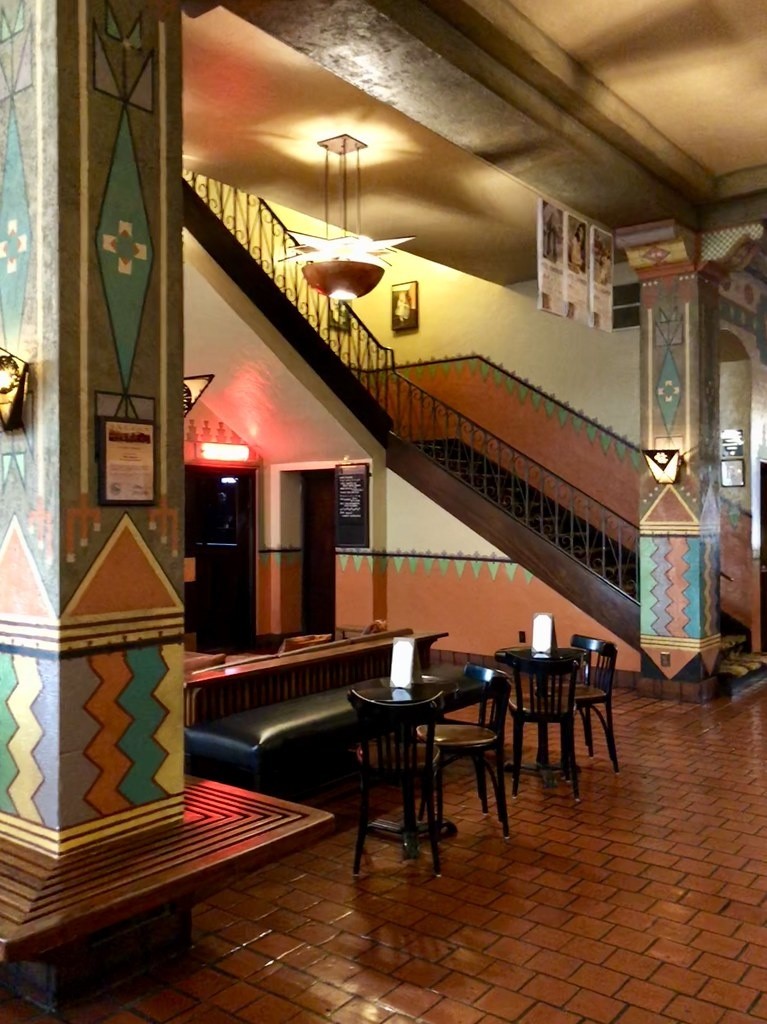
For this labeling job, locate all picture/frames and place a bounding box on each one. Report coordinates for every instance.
[98,417,157,507]
[391,280,418,330]
[721,459,745,487]
[327,297,352,330]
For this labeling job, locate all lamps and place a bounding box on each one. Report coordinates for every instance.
[641,449,683,484]
[278,136,415,300]
[183,374,214,417]
[0,346,30,431]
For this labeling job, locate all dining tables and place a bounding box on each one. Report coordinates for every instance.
[352,675,460,858]
[495,646,585,787]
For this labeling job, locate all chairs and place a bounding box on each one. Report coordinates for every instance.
[416,662,511,840]
[347,688,444,879]
[534,634,620,783]
[506,652,581,802]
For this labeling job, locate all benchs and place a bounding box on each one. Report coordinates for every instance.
[185,621,414,676]
[184,633,511,802]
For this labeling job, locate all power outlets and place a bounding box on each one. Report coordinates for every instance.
[661,652,671,666]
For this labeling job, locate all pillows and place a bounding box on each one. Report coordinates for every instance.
[278,634,332,653]
[184,653,226,673]
